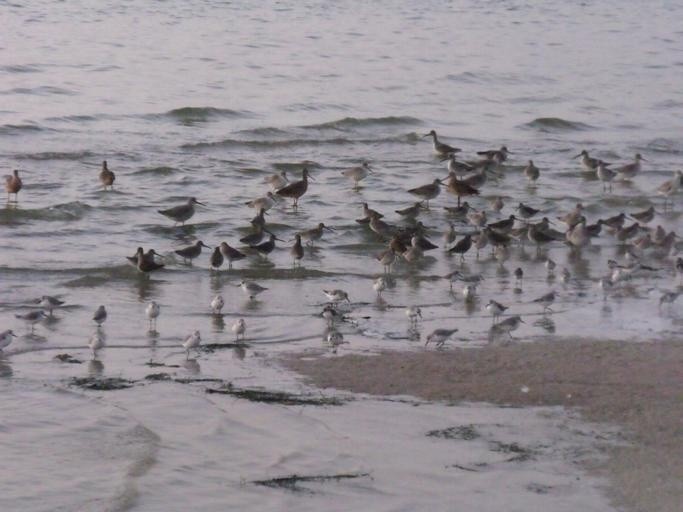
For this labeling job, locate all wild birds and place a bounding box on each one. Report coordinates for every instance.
[14,310,48,333]
[98,160,116,192]
[124,145,683,361]
[87,327,105,360]
[91,304,108,328]
[35,295,66,316]
[2,169,22,202]
[422,129,463,157]
[0,328,19,353]
